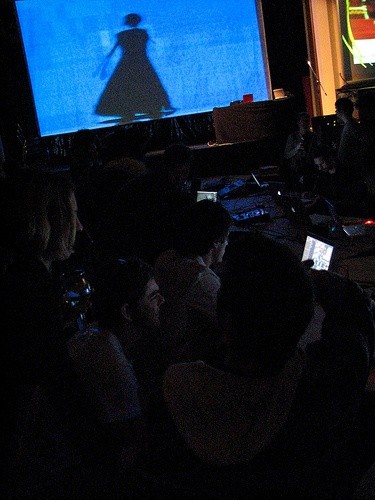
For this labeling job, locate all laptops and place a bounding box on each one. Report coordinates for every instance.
[300,231,336,273]
[195,191,218,202]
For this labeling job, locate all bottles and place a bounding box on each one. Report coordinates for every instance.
[53,266,92,330]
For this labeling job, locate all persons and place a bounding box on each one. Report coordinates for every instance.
[106,260,171,489]
[335,97,369,217]
[55,114,375,441]
[96,14,174,122]
[0,169,125,500]
[162,240,313,500]
[296,326,375,500]
